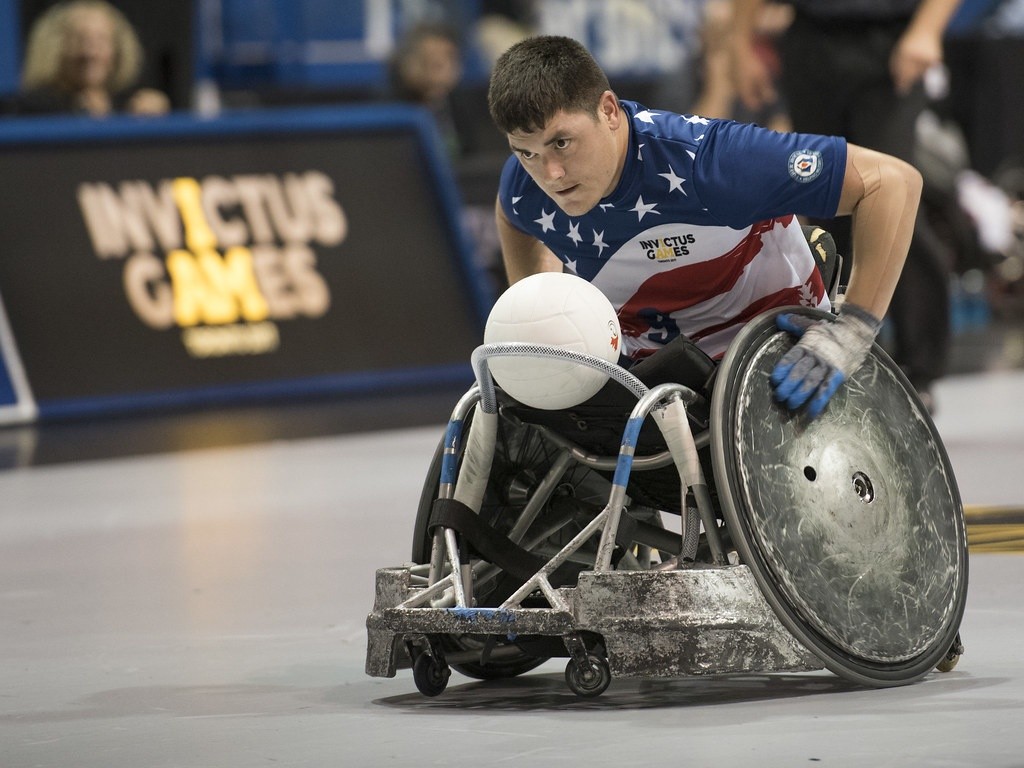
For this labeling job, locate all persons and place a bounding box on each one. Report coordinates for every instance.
[391,15,529,263]
[656,1,1024,413]
[1,0,139,116]
[490,37,923,414]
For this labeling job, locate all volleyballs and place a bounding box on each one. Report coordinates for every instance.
[481,272,624,413]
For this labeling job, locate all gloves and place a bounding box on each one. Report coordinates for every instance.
[771,300,884,419]
